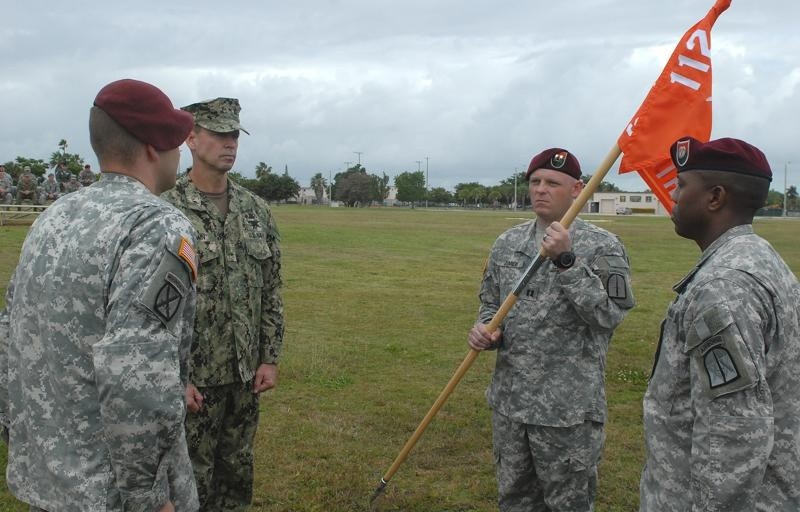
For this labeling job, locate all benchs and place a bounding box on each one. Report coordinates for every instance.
[0,204,49,225]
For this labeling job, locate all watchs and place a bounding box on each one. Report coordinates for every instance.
[552,247,576,269]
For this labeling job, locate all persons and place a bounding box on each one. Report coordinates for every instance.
[465,149,635,511]
[158,97,285,512]
[0,162,96,211]
[1,78,196,512]
[638,135,800,512]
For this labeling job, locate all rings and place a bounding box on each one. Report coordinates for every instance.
[543,234,549,241]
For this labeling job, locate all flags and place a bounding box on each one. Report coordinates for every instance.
[619,0,732,215]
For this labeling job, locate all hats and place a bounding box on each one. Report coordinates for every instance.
[93,78,196,151]
[670,135,773,181]
[525,148,582,181]
[179,97,252,136]
